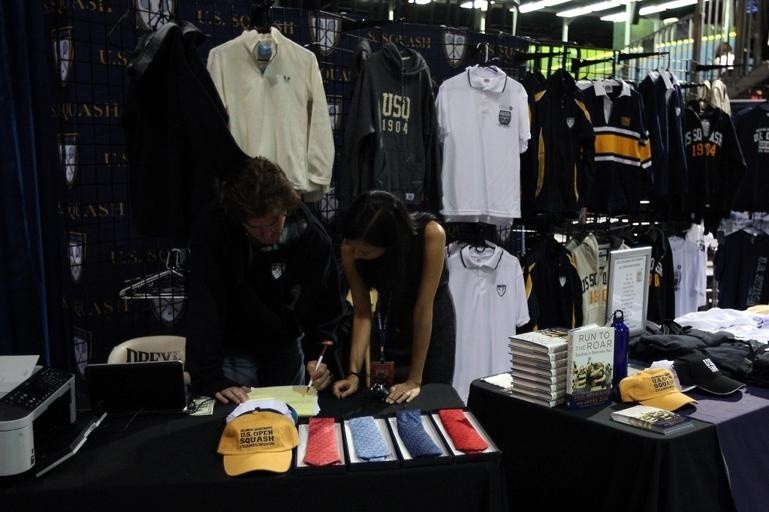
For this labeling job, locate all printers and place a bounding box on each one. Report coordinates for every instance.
[0,366,108,476]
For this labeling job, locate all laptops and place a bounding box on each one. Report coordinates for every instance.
[84,363,187,414]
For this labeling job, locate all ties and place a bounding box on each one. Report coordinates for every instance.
[303,417,342,468]
[348,416,391,462]
[396,408,443,458]
[438,408,489,454]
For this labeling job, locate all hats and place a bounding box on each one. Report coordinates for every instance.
[216,397,298,476]
[618,347,747,411]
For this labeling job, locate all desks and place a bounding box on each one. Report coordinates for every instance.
[470,363,769,512]
[0,383,502,512]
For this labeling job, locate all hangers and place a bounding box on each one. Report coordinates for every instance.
[118,247,194,301]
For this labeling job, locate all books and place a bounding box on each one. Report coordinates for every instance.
[507,322,615,411]
[611,404,695,436]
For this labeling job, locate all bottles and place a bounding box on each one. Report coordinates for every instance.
[610,309,630,395]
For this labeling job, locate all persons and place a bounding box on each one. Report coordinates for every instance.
[331,187,456,407]
[186,159,347,405]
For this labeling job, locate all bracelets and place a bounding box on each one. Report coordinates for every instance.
[345,371,360,379]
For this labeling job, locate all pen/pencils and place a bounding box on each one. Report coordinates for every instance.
[306,345,328,394]
